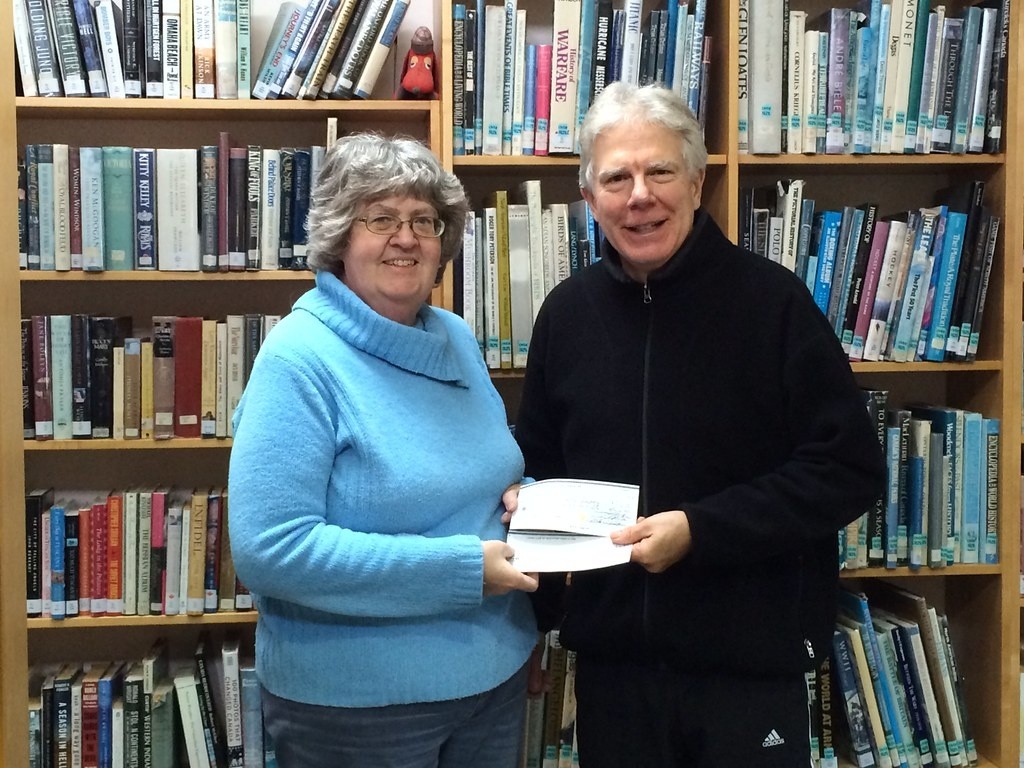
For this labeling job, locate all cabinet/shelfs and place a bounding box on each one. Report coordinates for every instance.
[0,0,1024,768]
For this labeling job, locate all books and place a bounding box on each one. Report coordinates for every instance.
[19,1,1002,767]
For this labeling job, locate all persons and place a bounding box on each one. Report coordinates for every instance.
[228,134,540,768]
[504,80,887,768]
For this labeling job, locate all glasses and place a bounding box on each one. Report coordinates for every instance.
[354,214,446,237]
